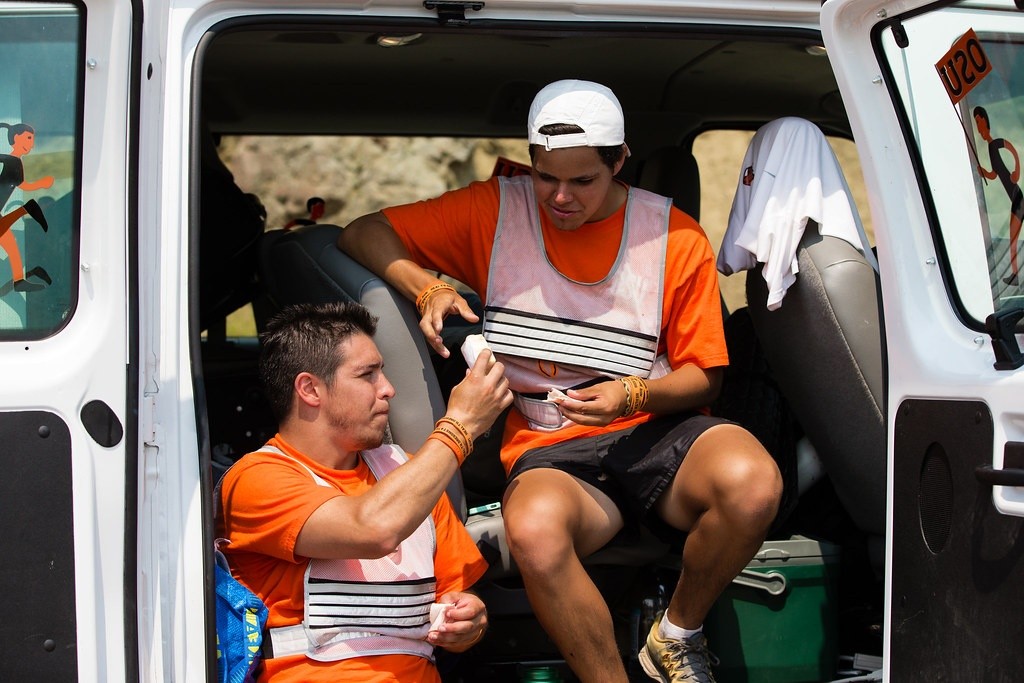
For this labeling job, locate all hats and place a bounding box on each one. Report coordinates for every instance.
[527,79,631,158]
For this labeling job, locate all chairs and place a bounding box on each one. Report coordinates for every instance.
[716,110,890,525]
[279,221,670,575]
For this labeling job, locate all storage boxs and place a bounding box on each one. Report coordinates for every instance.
[649,534,828,683]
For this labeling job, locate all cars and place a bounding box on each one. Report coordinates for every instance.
[0,0,1024,683]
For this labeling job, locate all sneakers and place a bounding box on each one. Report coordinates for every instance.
[638,609,721,683]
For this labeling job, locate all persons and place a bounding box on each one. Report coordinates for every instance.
[339,79,784,683]
[212,302,514,683]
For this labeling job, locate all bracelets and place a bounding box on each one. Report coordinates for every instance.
[415,283,455,316]
[616,376,649,418]
[427,416,474,468]
